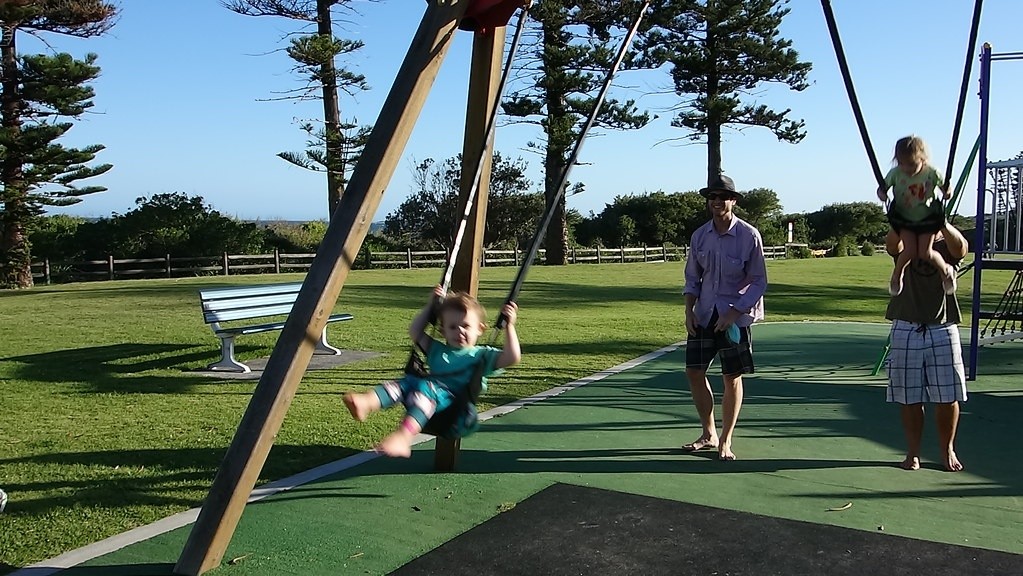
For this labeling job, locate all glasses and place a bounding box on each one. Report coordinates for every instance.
[706,193,731,201]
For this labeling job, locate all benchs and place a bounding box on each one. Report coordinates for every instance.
[199,284,354,373]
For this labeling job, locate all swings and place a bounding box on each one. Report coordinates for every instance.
[401,0,652,438]
[819,0,985,242]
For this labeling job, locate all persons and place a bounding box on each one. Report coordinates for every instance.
[877,136,956,298]
[684,176,768,462]
[344,282,521,459]
[885,214,970,471]
[985,239,997,259]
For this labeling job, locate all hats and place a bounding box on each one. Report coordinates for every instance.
[700,175,743,200]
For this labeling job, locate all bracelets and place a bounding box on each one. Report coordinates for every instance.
[731,305,741,314]
